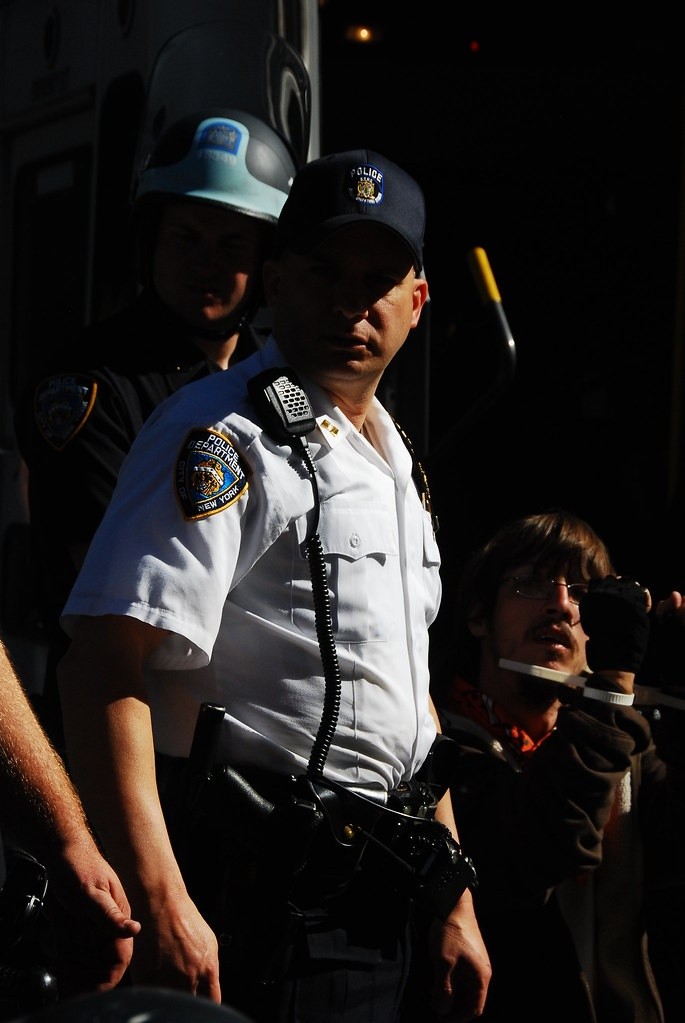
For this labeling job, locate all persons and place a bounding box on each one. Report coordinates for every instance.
[13,104,306,756]
[430,514,685,1022]
[1,638,142,995]
[58,149,497,1023]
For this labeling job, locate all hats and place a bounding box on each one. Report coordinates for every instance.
[278,148,426,272]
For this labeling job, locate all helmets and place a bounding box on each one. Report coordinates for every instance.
[134,107,299,224]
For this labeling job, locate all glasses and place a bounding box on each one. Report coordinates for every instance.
[501,574,588,605]
